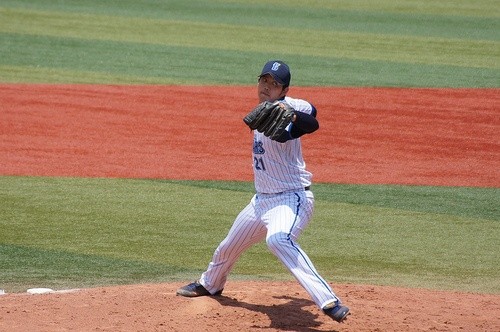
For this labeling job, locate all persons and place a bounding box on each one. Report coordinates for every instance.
[178,60,351,321]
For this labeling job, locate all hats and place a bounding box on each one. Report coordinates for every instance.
[258,60,291,84]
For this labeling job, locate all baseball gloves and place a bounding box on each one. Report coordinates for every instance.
[241,100,295,141]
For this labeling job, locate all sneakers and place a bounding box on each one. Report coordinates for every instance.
[177,280,224,297]
[323,302,350,322]
